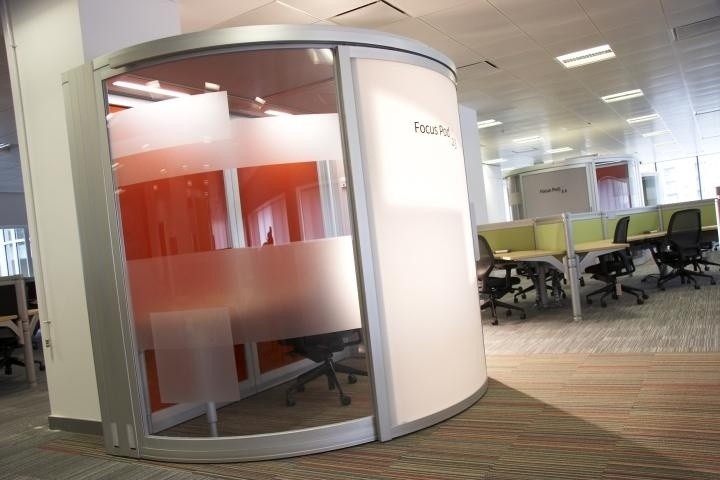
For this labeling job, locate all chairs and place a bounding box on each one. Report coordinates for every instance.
[277,328,368,407]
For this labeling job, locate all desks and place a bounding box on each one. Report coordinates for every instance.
[0,274,46,389]
[477,198,720,326]
